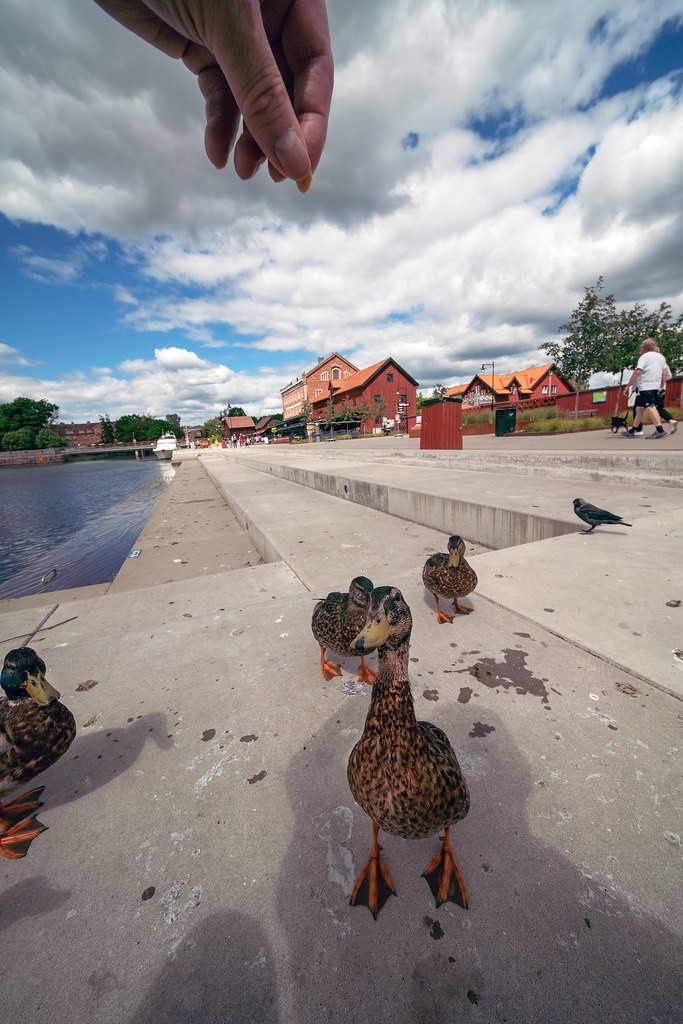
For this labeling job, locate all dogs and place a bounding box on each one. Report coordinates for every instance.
[611,412,629,432]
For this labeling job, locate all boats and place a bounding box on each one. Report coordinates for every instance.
[153,429,182,459]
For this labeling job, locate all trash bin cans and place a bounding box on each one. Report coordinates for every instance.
[417,396,463,450]
[495,405,516,437]
[222,440,227,448]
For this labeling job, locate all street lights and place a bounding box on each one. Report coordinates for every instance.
[396,387,408,434]
[480,361,494,410]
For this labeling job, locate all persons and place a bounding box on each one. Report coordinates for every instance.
[239,433,244,446]
[246,434,250,448]
[251,434,255,445]
[222,438,226,448]
[233,434,237,448]
[621,339,668,439]
[93,0,335,183]
[634,347,678,435]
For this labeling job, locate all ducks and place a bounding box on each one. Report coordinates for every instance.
[348,583,469,920]
[0,646,76,859]
[421,535,478,624]
[311,575,376,685]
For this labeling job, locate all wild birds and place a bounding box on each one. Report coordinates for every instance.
[572,497,632,535]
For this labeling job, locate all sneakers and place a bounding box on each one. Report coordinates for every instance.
[633,429,643,436]
[645,431,667,439]
[621,430,633,438]
[669,420,678,434]
